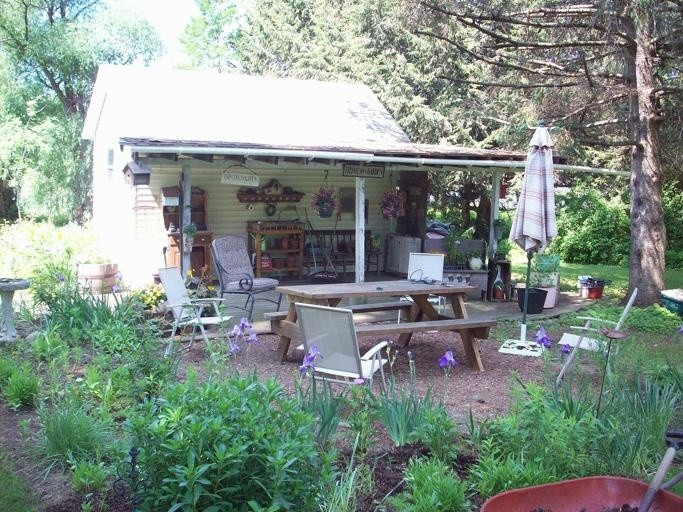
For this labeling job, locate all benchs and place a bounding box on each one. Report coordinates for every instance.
[304,229,383,278]
[354,317,498,373]
[264,301,414,363]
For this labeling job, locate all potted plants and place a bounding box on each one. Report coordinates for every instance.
[581,277,612,299]
[183,223,198,254]
[466,240,560,309]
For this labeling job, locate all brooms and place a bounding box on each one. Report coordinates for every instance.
[303,208,338,279]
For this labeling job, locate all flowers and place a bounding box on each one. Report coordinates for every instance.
[309,184,337,209]
[377,190,407,221]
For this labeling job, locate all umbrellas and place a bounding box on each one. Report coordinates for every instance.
[498,122,556,357]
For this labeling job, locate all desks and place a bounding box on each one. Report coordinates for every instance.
[274,280,486,374]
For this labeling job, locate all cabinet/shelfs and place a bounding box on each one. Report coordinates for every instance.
[246,221,305,280]
[159,185,214,277]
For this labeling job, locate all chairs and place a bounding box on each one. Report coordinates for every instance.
[555,287,639,389]
[157,266,237,364]
[208,234,283,322]
[294,301,388,394]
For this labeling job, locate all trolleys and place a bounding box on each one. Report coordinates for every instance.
[473,467,682,511]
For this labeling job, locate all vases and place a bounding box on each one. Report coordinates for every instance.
[517,288,548,313]
[318,200,334,218]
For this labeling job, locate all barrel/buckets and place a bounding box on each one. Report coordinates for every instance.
[588,288,602,299]
